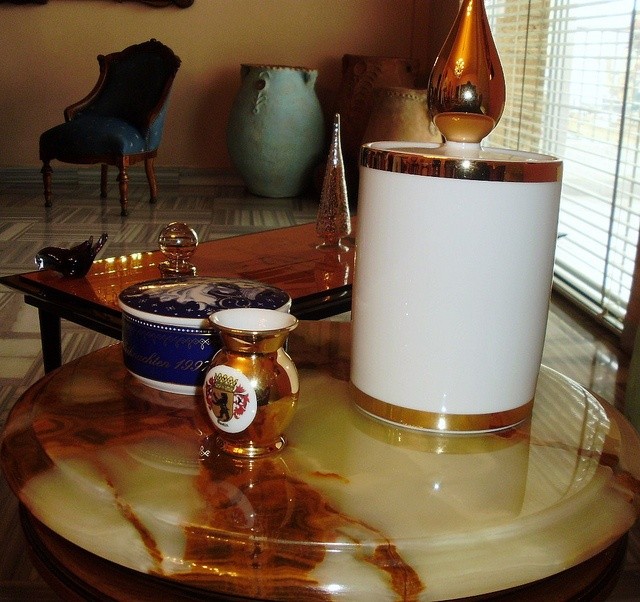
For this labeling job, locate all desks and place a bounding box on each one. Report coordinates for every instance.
[0,317,640,602]
[0,217,357,375]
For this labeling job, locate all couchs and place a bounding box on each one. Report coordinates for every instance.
[39,38,182,218]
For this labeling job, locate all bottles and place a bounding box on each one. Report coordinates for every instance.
[199,307,300,460]
